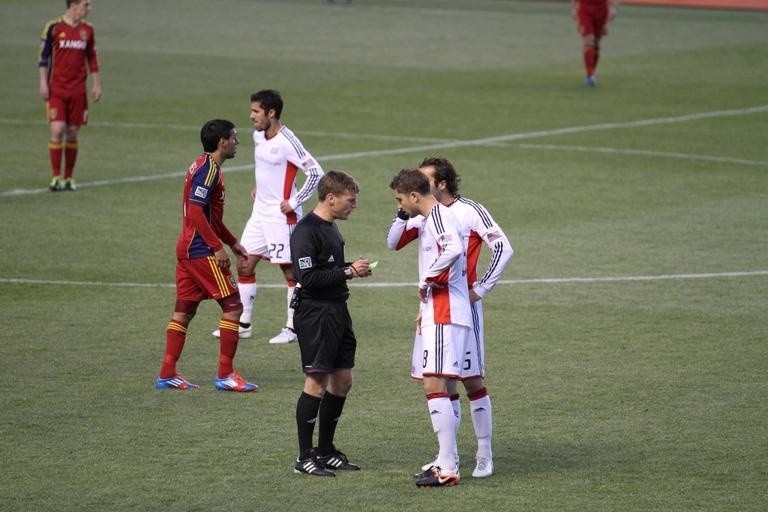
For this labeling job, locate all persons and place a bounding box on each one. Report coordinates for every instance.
[290,171,372,478]
[155,119,259,393]
[212,90,327,344]
[386,157,514,477]
[389,168,471,488]
[571,1,615,86]
[39,0,101,191]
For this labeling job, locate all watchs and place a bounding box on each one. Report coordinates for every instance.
[344,267,353,278]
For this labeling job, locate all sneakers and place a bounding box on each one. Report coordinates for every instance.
[49,177,63,191]
[294,447,336,476]
[212,322,252,339]
[471,455,494,478]
[214,371,258,392]
[155,375,200,391]
[316,450,361,471]
[587,76,597,87]
[416,466,460,489]
[414,466,460,481]
[63,179,77,191]
[269,327,298,344]
[421,456,460,470]
